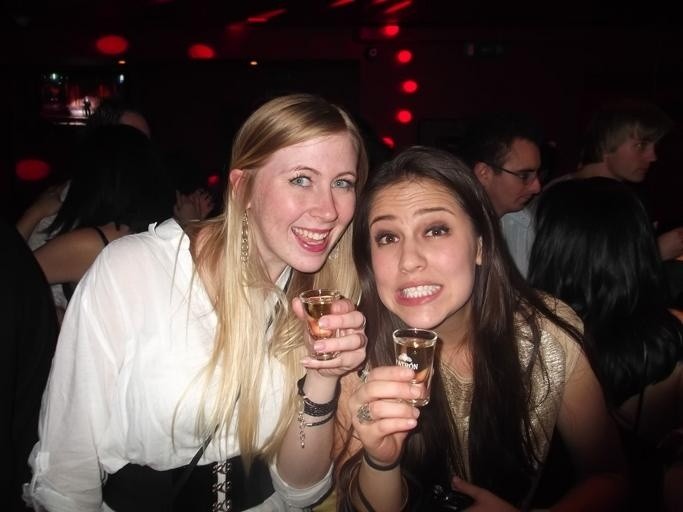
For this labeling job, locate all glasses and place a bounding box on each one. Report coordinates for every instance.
[490,164,543,186]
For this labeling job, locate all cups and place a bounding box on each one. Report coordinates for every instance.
[392,328,438,407]
[299,288,341,361]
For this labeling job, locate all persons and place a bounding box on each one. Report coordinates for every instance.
[0,77,681,512]
